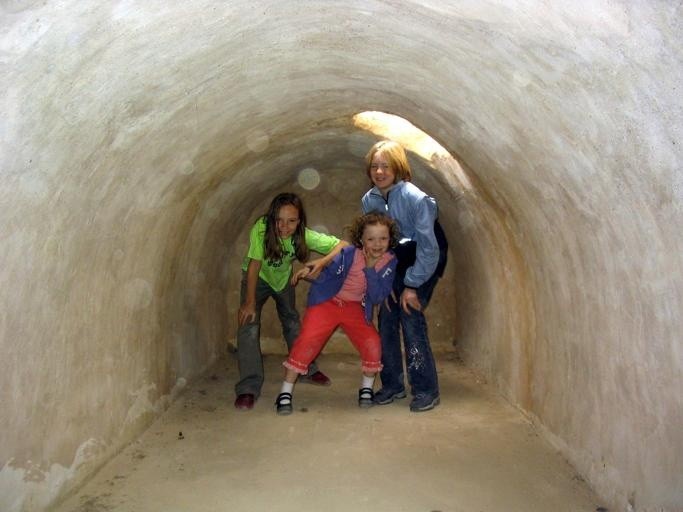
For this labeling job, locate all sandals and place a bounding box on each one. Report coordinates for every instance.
[359,388,373,406]
[275,393,292,414]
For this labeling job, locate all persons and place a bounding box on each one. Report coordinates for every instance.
[359,139,449,413]
[232,192,352,410]
[273,210,399,416]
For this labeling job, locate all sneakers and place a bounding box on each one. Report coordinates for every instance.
[296,371,331,386]
[373,385,406,404]
[410,390,440,412]
[235,394,254,410]
